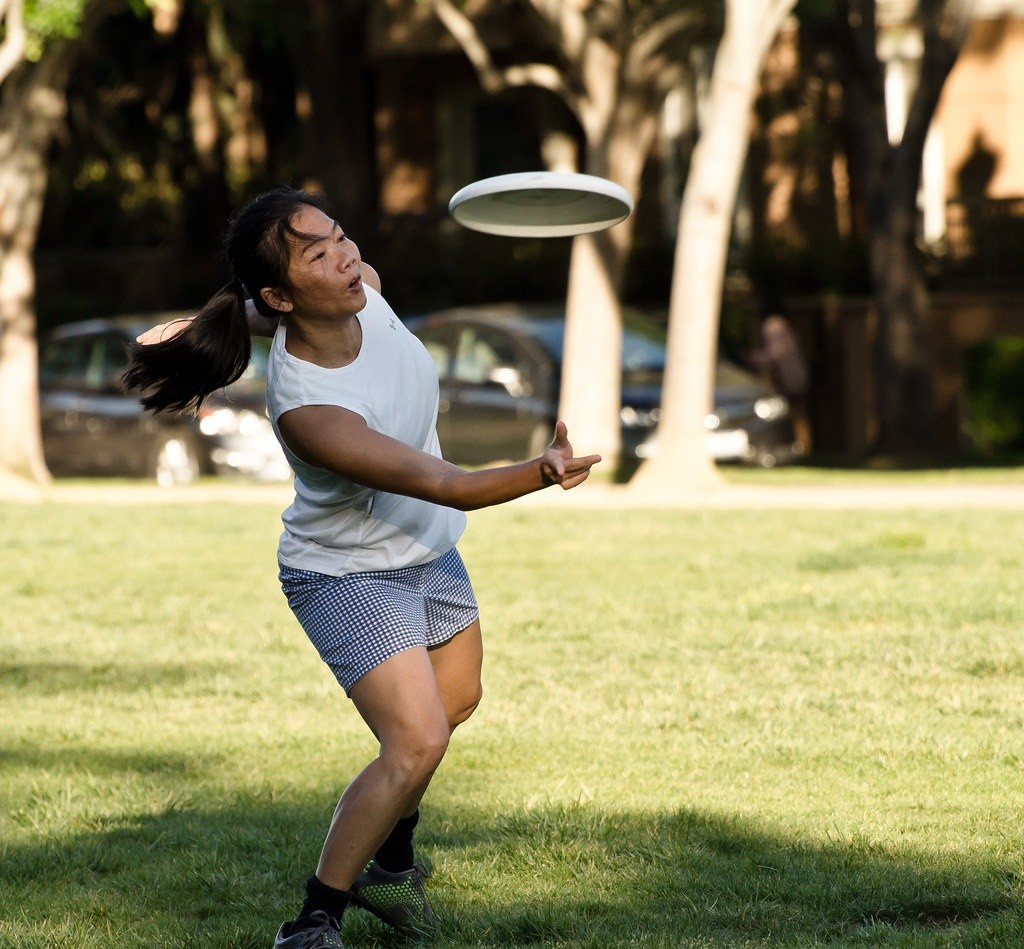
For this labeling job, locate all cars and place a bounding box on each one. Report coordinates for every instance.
[395,298,797,468]
[33,311,274,485]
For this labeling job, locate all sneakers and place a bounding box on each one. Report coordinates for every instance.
[346,858,450,946]
[270,909,347,949]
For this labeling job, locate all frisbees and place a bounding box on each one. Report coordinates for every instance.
[448,171,634,237]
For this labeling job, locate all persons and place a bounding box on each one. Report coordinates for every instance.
[117,194,603,949]
